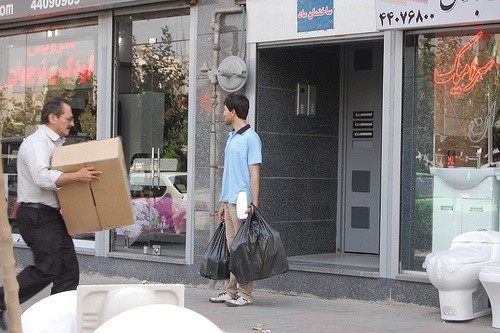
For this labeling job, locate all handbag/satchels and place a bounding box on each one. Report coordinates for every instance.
[199,221,230,281]
[229,202,289,287]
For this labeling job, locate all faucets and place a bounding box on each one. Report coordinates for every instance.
[464,145,482,169]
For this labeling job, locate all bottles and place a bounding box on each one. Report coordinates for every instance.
[447,150,456,168]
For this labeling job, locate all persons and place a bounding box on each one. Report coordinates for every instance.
[0,99,102,330]
[209,95,263,306]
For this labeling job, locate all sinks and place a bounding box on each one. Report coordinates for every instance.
[429,167,500,190]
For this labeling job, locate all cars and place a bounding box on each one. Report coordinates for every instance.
[128,171,187,200]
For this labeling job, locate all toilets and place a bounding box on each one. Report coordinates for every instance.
[426,227,500,321]
[478,260,500,329]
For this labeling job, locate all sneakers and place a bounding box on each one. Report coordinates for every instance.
[209,290,254,308]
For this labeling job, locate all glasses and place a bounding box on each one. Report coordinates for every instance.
[56,114,78,124]
